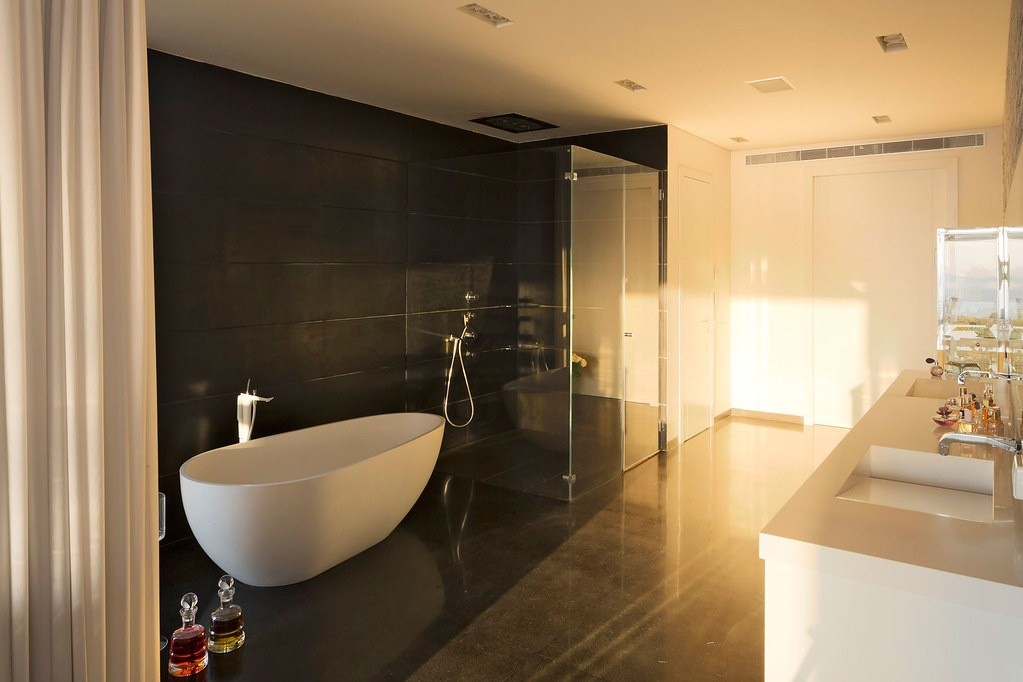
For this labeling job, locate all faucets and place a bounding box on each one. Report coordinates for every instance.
[235,379,275,443]
[957,371,1011,385]
[938,432,1019,457]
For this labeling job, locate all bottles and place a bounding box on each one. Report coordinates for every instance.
[929,361,943,377]
[168,574,246,677]
[933,386,1003,432]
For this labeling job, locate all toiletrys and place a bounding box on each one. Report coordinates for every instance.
[208,575,246,654]
[931,388,1004,436]
[168,591,208,677]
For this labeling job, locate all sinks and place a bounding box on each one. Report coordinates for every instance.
[834,446,995,523]
[904,378,993,401]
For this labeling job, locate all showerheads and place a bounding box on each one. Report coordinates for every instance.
[460,325,471,340]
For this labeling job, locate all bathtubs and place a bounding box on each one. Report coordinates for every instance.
[501,367,658,466]
[179,412,446,586]
